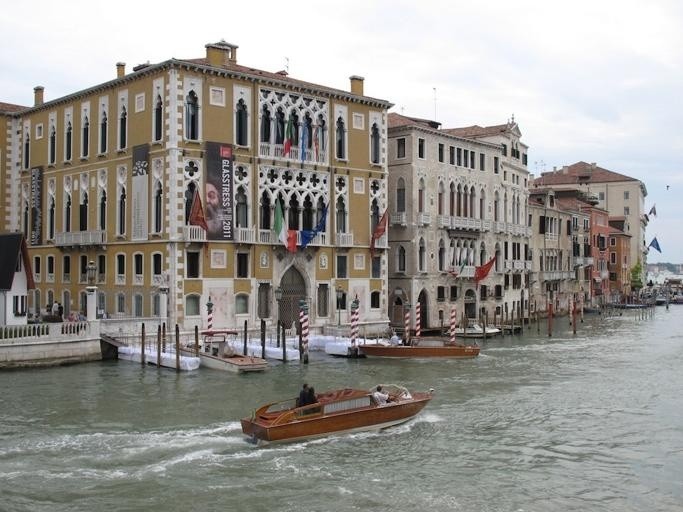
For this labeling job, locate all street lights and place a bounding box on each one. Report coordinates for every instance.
[87,260,97,286]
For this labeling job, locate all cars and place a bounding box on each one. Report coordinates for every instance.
[27,313,42,324]
[62,313,87,334]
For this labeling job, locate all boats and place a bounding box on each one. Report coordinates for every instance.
[173,330,267,376]
[357,335,480,357]
[239,383,436,446]
[607,294,683,308]
[443,324,521,338]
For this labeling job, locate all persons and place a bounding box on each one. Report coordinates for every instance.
[388,332,399,346]
[53,301,64,322]
[373,385,390,405]
[299,384,320,415]
[206,182,223,235]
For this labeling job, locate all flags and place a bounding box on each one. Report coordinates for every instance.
[274,199,329,252]
[189,190,208,231]
[283,118,319,162]
[650,238,661,253]
[649,207,656,216]
[370,211,388,258]
[475,257,495,281]
